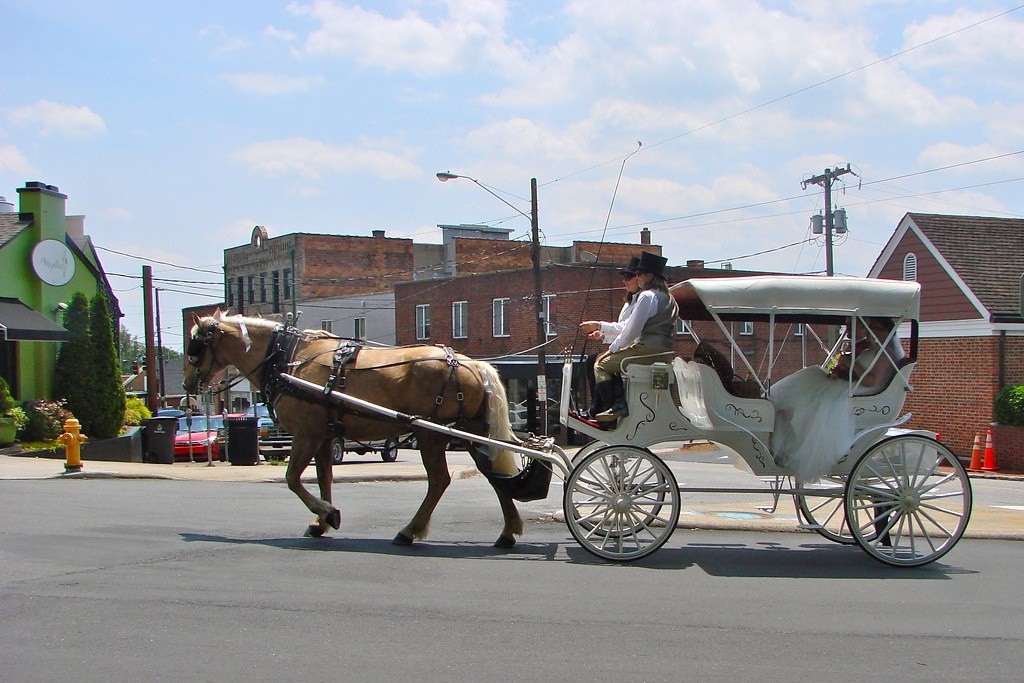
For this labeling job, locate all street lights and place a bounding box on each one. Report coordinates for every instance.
[435,171,549,439]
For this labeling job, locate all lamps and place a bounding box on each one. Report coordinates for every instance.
[50,303,68,314]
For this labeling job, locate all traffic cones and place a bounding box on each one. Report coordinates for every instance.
[981,429,1000,471]
[964,431,985,473]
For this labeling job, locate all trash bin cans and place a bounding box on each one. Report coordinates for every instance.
[141,416,179,465]
[223,417,260,466]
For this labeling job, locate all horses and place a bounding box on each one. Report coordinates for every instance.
[181,306,523,549]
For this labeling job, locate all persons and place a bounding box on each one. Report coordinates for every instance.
[762,316,905,486]
[577,251,680,430]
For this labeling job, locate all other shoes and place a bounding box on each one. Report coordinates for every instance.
[588,406,596,419]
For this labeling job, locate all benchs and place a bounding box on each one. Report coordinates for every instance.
[671,342,774,433]
[849,357,918,430]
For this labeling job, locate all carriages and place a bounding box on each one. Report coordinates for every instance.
[182,266,975,571]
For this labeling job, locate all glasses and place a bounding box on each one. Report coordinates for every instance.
[636,272,647,276]
[624,273,635,281]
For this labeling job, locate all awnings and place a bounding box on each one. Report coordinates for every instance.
[0,297,70,342]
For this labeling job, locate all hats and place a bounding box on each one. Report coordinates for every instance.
[635,251,669,281]
[617,256,640,273]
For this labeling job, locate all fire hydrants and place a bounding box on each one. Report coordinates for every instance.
[57,418,88,472]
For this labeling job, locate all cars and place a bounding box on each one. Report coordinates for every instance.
[156,400,464,465]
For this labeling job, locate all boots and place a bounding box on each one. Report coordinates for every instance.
[596,380,629,422]
[597,381,616,429]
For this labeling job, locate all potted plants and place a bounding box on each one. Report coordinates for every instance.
[0,406,30,448]
